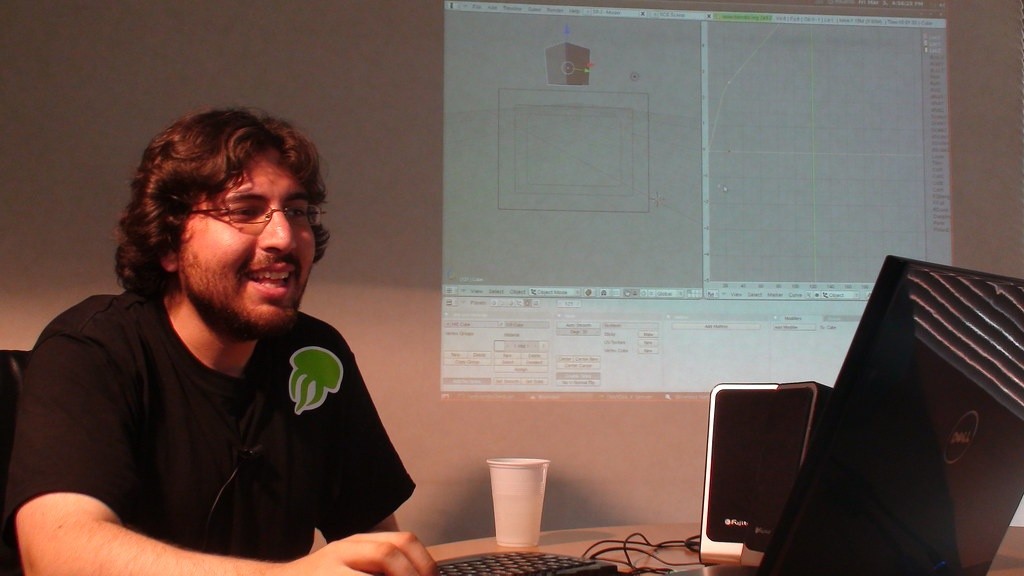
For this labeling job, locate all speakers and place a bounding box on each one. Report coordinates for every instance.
[698,381,835,569]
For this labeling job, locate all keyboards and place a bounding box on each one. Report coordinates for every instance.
[433,551,617,576]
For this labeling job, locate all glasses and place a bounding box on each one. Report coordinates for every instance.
[190,199,326,223]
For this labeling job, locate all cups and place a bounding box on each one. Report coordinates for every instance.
[486,458,551,547]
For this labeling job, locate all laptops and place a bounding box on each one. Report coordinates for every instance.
[667,255,1024,576]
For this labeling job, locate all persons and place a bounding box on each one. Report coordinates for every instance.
[6,109,439,576]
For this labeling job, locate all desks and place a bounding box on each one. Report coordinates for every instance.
[427,524,1024,576]
